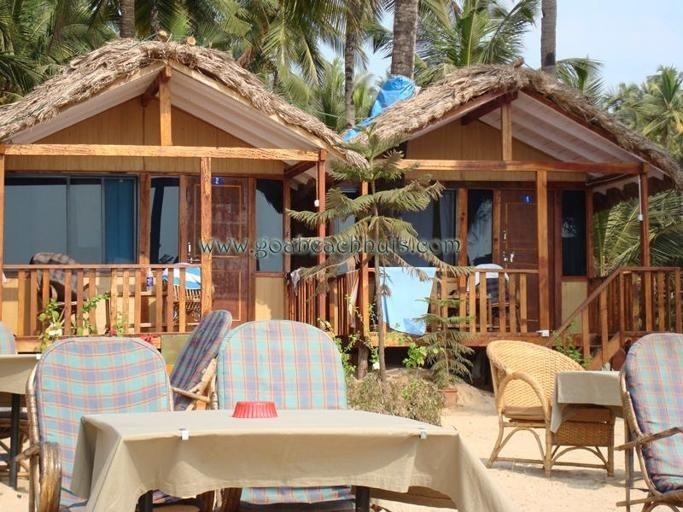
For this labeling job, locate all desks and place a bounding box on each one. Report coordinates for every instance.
[439,293,490,330]
[84,409,459,512]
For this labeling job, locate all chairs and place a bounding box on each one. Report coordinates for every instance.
[485,331,683,512]
[0,311,373,512]
[468,263,522,332]
[31,252,216,335]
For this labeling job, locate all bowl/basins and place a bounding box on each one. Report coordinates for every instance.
[231,402,279,418]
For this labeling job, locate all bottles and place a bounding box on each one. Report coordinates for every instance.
[144,267,154,292]
